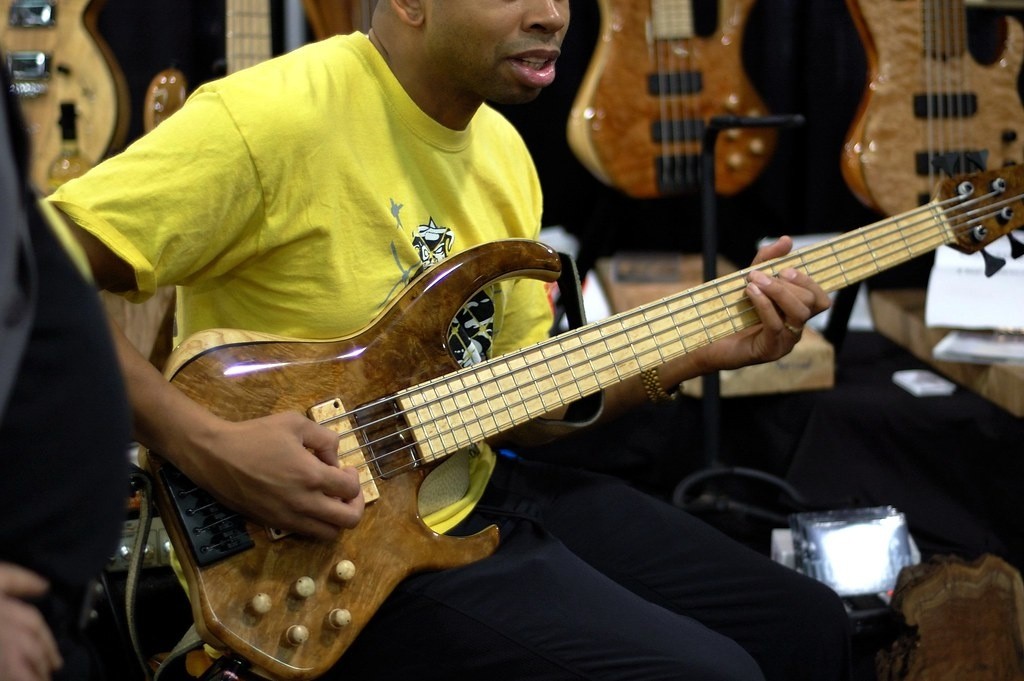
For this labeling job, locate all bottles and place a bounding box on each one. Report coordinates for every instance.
[46,101,96,198]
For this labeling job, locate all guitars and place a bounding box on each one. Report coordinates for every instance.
[151,164,1024,680]
[564,1,779,201]
[0,0,132,196]
[130,0,275,145]
[834,0,1024,219]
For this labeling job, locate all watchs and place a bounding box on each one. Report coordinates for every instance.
[641,367,680,404]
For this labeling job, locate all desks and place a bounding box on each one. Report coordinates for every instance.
[868,283,1024,422]
[557,257,841,409]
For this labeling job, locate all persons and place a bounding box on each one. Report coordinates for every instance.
[0,57,129,681]
[43,0,850,681]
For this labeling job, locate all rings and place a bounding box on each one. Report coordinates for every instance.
[785,322,803,337]
